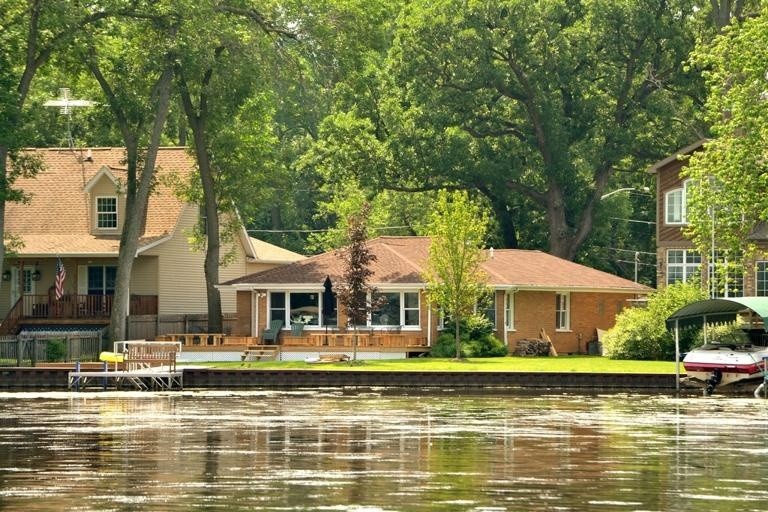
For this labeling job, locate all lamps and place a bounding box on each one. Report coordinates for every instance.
[30,269,42,281]
[1,269,12,282]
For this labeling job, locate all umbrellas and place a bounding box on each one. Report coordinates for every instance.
[320,273,336,344]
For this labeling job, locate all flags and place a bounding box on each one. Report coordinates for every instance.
[54,258,66,302]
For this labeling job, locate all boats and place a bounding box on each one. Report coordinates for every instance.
[681,340,768,398]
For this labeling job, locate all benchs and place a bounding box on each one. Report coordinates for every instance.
[122,343,180,373]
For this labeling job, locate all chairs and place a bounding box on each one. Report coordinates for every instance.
[718,346,746,351]
[261,319,284,345]
